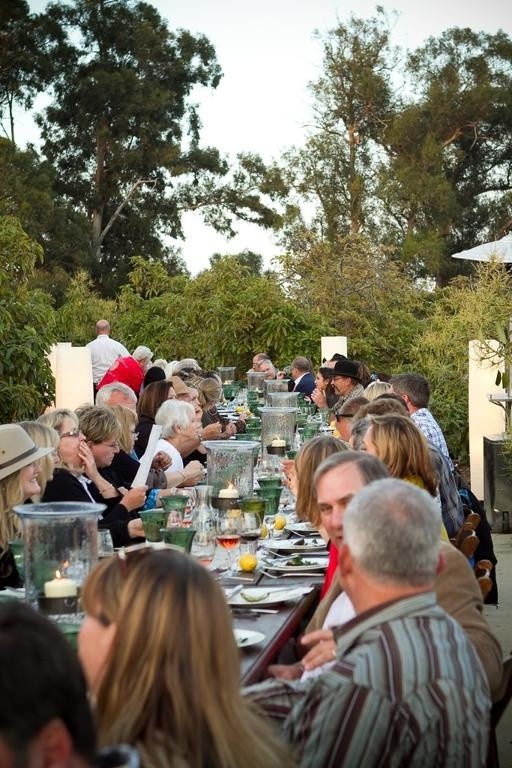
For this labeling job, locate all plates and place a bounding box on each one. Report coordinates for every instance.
[263,521,329,573]
[222,587,302,605]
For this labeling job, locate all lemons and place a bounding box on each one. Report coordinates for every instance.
[239,555,257,571]
[275,517,285,530]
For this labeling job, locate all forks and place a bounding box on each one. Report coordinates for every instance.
[259,569,327,579]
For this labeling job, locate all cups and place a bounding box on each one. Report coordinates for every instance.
[212,365,338,455]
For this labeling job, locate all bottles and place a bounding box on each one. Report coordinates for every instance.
[10,439,283,657]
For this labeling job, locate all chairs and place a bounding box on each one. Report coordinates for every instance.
[451,462,512,767]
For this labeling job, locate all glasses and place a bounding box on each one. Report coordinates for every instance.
[334,411,356,423]
[60,428,79,438]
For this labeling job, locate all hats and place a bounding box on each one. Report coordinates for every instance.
[328,360,359,380]
[0,423,55,481]
[167,375,190,396]
